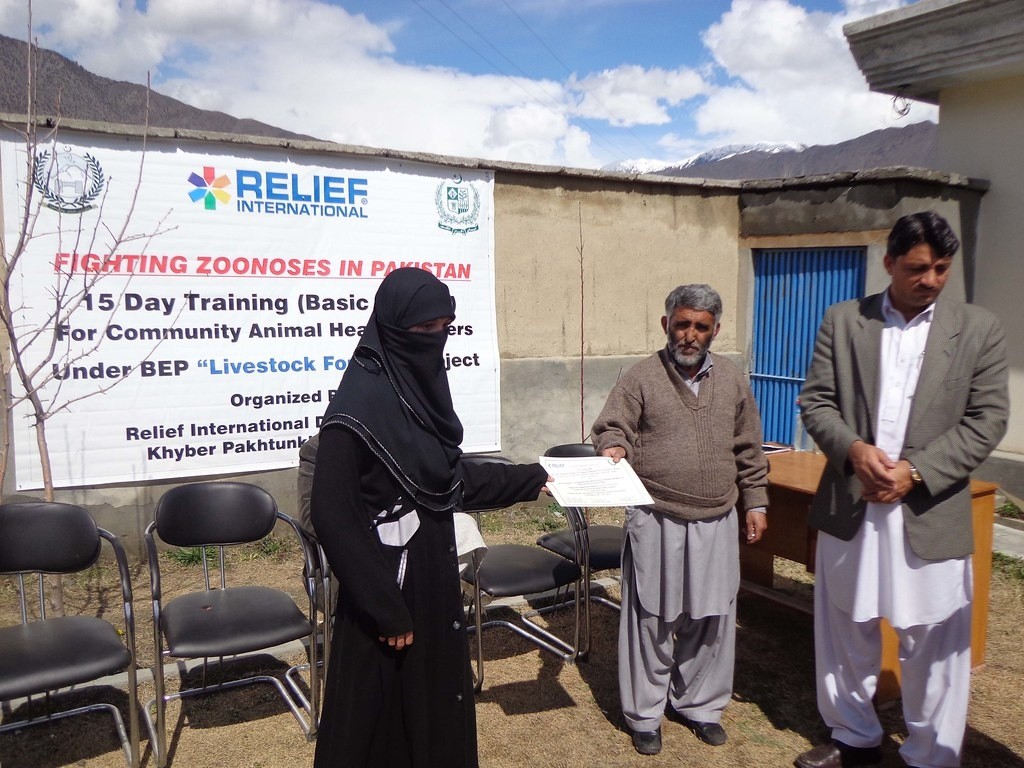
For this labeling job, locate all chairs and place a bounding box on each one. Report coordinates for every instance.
[458,454,582,691]
[141,481,318,768]
[0,503,141,768]
[520,443,623,658]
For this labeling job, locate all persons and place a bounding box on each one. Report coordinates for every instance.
[298,267,554,768]
[797,211,1011,768]
[591,284,771,755]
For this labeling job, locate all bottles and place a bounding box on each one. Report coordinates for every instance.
[794,394,814,451]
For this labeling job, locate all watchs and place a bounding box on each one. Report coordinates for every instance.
[910,462,922,486]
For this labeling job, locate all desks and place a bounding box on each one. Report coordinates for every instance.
[738,441,1000,706]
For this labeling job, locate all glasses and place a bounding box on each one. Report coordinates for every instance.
[670,320,712,332]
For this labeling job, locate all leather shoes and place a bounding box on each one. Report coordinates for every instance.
[633,725,662,754]
[664,698,727,746]
[796,741,883,768]
